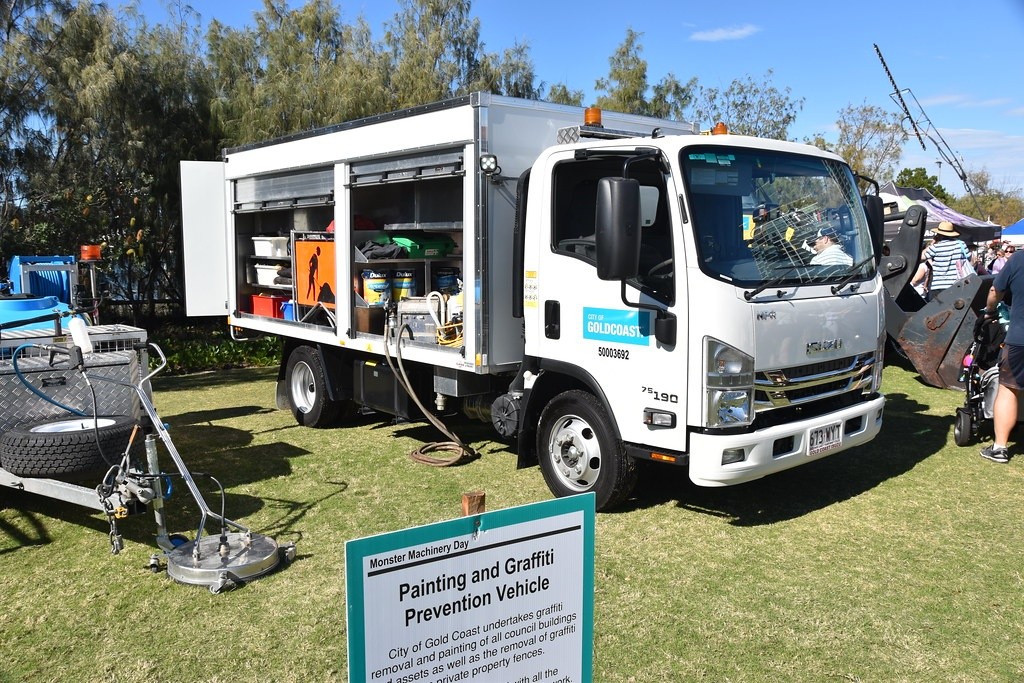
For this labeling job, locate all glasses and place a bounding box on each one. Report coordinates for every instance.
[1005,250,1014,253]
[996,253,1001,256]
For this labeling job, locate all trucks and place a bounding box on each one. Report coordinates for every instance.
[178,89,890,516]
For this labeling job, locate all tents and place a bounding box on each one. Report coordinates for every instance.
[872,181,1024,265]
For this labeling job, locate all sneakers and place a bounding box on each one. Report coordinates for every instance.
[980,443,1009,463]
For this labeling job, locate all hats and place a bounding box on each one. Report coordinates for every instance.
[810,226,840,241]
[931,220,960,237]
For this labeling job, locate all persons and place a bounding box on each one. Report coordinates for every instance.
[810,225,853,266]
[910,222,1017,303]
[980,248,1024,462]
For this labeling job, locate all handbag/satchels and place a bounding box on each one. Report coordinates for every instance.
[987,258,997,270]
[979,366,1024,421]
[956,239,978,280]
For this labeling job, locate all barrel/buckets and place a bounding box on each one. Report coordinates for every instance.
[363,268,391,310]
[392,268,415,316]
[281,301,299,321]
[435,267,460,295]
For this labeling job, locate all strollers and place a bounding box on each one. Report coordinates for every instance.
[953,307,1010,447]
